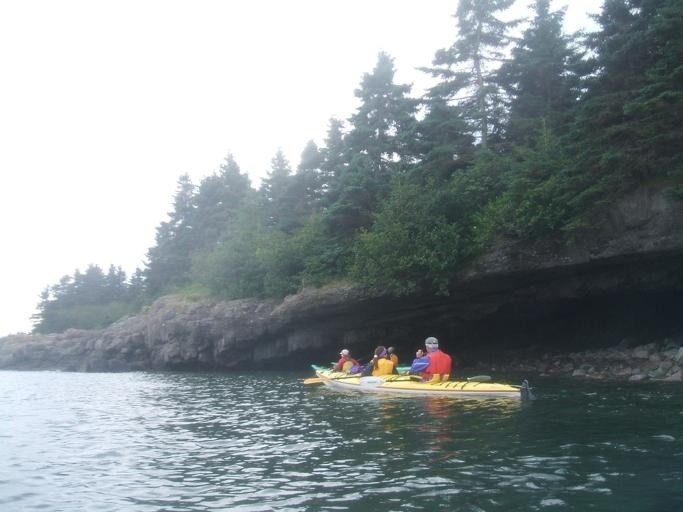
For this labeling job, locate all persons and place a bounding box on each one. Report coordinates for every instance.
[386,346,400,367]
[332,348,360,374]
[360,345,400,378]
[408,336,453,383]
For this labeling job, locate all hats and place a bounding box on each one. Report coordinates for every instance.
[340,349,349,355]
[425,337,439,344]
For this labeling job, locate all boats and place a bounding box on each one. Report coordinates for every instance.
[312,364,535,398]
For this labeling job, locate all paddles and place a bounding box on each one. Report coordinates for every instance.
[303,376,363,385]
[361,376,491,386]
